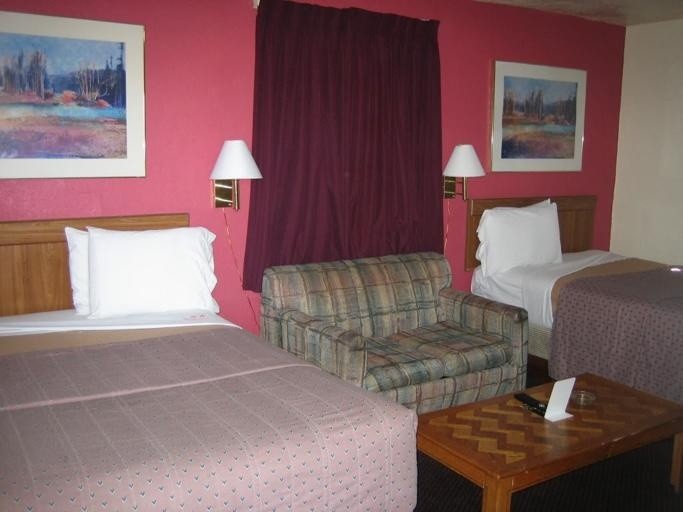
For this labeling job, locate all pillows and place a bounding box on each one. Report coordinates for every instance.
[471,196,567,279]
[63,223,222,319]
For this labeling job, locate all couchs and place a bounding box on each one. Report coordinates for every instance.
[260,249,530,415]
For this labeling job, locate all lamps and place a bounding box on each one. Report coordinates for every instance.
[208,139,264,208]
[441,144,487,202]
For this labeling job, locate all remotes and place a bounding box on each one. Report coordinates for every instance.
[514,392,547,411]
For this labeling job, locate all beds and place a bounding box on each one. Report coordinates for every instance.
[2,211,421,512]
[464,194,683,402]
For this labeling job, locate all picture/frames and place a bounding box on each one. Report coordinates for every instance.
[1,8,148,179]
[490,58,587,172]
